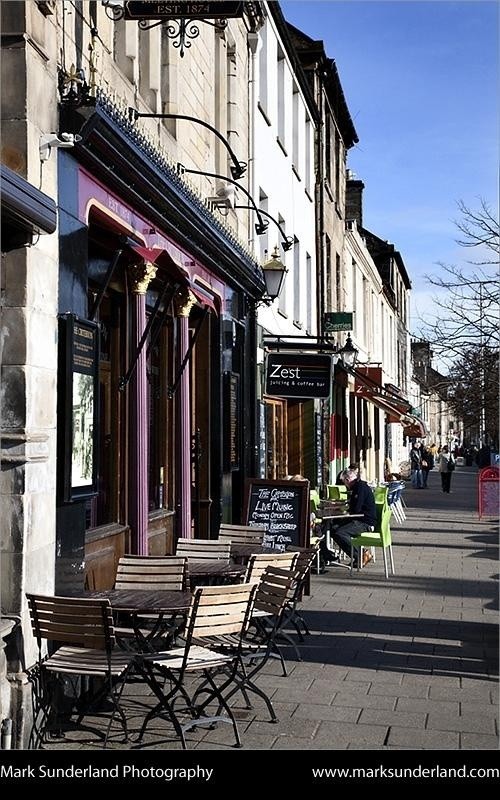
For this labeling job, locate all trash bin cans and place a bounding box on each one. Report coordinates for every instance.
[466,455,473,466]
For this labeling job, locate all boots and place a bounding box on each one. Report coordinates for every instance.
[359,549,373,568]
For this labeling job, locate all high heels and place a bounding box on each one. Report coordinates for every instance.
[325,555,339,563]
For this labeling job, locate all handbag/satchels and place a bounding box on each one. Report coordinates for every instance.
[448,461,455,471]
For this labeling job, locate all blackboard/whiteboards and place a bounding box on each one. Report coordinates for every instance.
[241,477,309,553]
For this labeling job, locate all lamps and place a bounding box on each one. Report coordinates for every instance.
[322,332,359,372]
[129,106,296,253]
[247,245,289,306]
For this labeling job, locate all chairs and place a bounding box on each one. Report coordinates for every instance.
[24,475,407,750]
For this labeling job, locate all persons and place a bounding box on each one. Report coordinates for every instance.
[334,463,360,485]
[289,474,339,574]
[328,470,376,569]
[409,441,470,489]
[438,445,456,495]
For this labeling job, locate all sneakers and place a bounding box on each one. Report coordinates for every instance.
[440,490,453,496]
